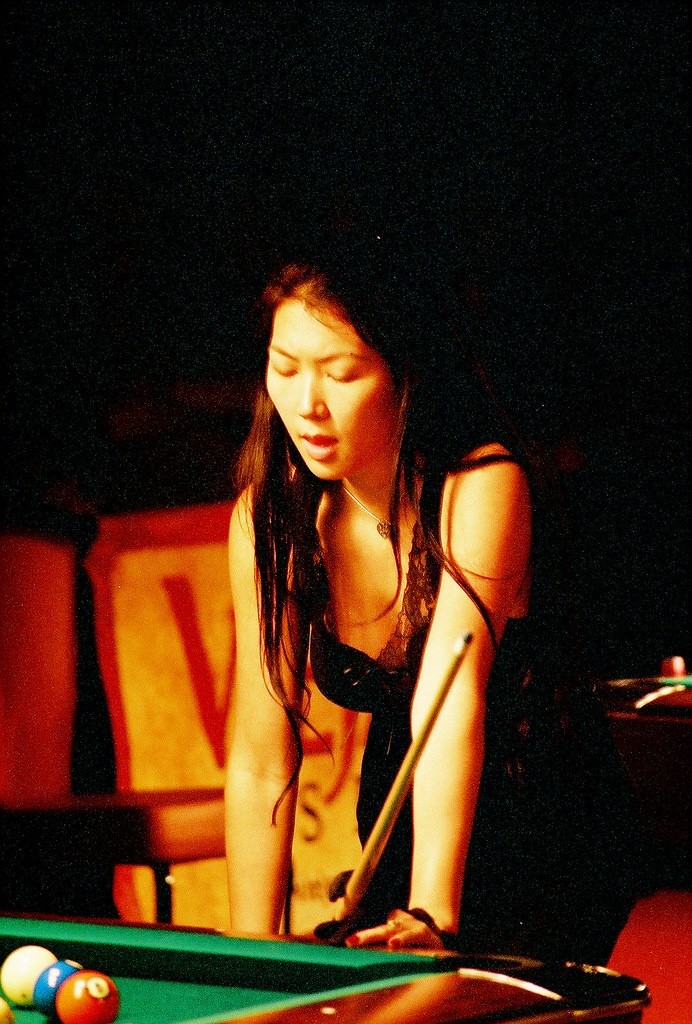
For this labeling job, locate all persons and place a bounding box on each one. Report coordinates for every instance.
[221,243,644,967]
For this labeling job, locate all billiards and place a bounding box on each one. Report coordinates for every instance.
[0,945,121,1024]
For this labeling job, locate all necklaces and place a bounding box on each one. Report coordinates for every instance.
[342,488,392,538]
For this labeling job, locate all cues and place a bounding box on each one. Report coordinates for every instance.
[336,631,473,921]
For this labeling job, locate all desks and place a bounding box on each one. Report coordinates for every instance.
[0,911,652,1024]
